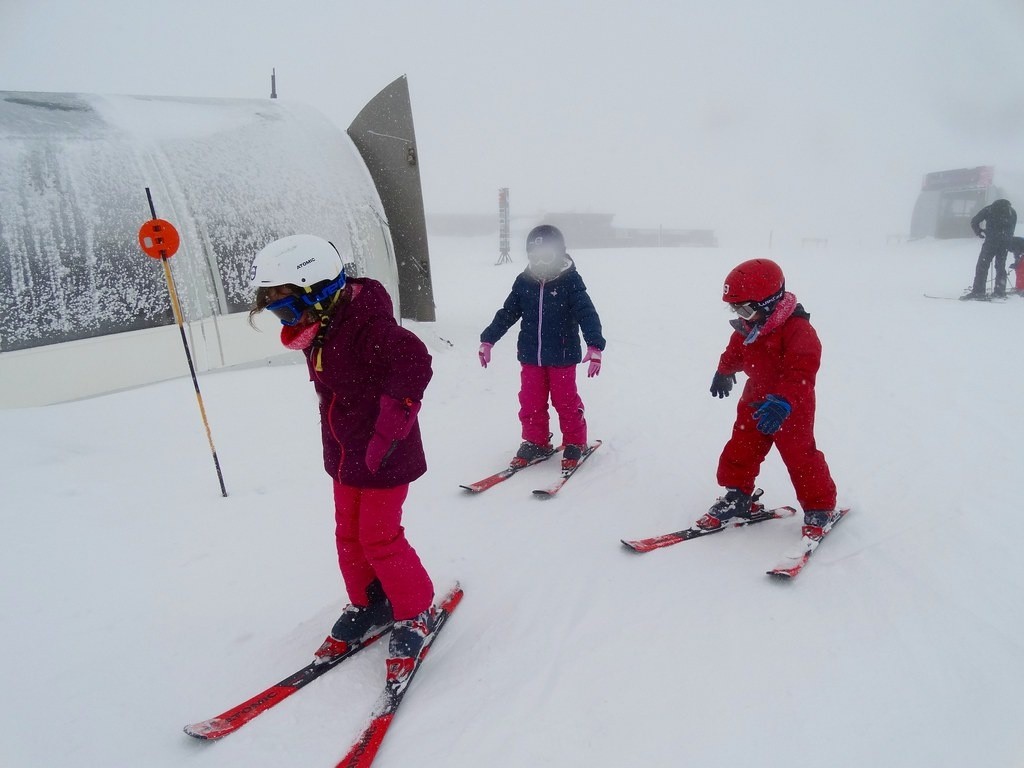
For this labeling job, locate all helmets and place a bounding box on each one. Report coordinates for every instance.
[250,234,343,287]
[526,225,566,274]
[723,259,784,303]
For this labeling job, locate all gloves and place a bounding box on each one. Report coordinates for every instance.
[582,345,602,376]
[709,370,733,398]
[366,397,422,474]
[752,395,791,436]
[478,340,493,368]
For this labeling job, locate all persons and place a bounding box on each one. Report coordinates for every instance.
[1006,237,1024,294]
[708,259,837,531]
[250,234,435,671]
[962,199,1017,299]
[478,225,607,474]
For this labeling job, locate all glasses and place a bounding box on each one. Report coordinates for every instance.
[734,302,758,320]
[528,249,555,264]
[268,296,304,326]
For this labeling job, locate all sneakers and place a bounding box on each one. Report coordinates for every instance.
[803,508,832,528]
[330,601,389,640]
[563,443,587,468]
[708,487,752,520]
[517,440,550,461]
[388,605,435,659]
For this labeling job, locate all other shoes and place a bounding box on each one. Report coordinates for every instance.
[961,292,983,300]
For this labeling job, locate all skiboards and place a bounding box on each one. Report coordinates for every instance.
[620,505,851,579]
[458,438,603,497]
[923,287,1024,303]
[180,580,465,768]
